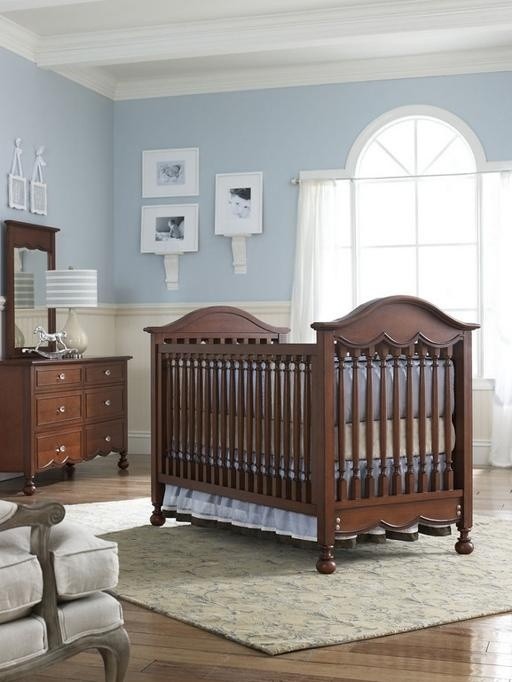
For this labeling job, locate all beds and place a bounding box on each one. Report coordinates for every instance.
[139,292,488,576]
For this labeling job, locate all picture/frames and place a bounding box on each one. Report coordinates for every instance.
[6,174,27,211]
[140,202,200,254]
[29,180,48,216]
[140,146,199,199]
[210,170,264,237]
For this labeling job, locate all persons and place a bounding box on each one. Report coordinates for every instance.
[161,165,181,183]
[229,188,251,219]
[168,219,182,239]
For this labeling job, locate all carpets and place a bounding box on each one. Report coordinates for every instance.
[27,496,512,659]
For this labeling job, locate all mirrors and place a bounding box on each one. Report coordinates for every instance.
[4,218,62,360]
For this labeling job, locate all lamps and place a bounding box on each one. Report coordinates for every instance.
[45,264,98,362]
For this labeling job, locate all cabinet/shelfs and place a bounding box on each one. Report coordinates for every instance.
[1,355,136,496]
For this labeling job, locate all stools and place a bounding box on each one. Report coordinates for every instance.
[1,496,132,680]
